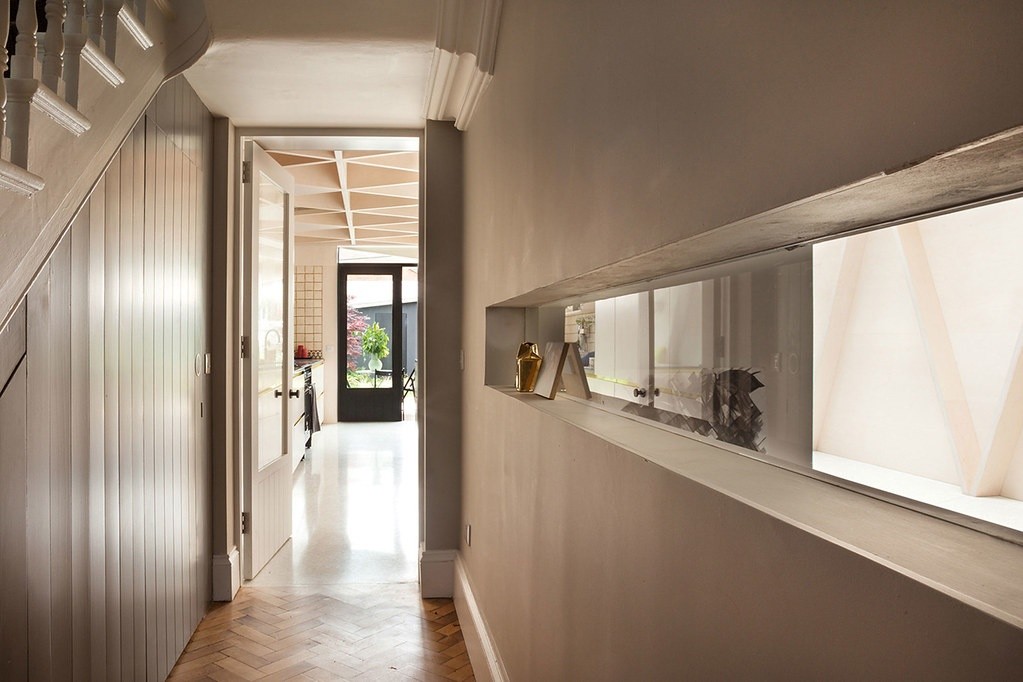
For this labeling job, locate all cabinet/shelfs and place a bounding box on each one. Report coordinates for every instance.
[293,373,307,472]
[310,363,325,425]
[594,275,731,425]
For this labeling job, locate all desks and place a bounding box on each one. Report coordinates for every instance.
[356,370,392,388]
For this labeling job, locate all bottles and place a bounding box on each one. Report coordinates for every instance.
[298,345,304,358]
[302,349,308,358]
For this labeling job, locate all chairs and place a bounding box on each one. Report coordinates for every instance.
[403,368,417,401]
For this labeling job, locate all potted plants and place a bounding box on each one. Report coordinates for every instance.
[352,321,389,371]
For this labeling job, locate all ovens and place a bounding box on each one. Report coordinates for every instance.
[304,366,313,435]
